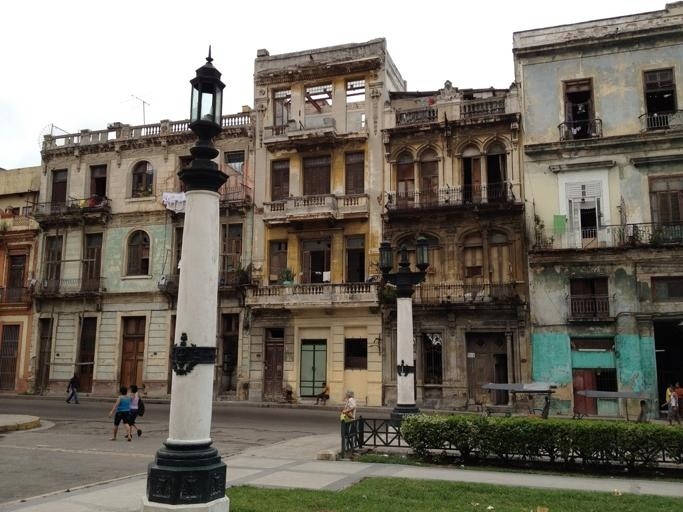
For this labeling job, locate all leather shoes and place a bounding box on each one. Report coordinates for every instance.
[125,434,132,438]
[137,429,142,436]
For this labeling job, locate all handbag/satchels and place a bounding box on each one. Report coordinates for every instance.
[136,399,145,417]
[340,412,354,423]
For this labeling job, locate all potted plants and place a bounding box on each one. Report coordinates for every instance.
[280,267,295,287]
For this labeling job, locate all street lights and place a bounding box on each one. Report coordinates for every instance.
[148,44,225,506]
[378,235,429,406]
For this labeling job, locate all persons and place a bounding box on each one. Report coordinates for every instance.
[65,372,81,404]
[124,385,142,438]
[636,400,652,424]
[314,383,330,405]
[96,196,108,207]
[341,391,356,438]
[107,387,132,441]
[661,383,683,426]
